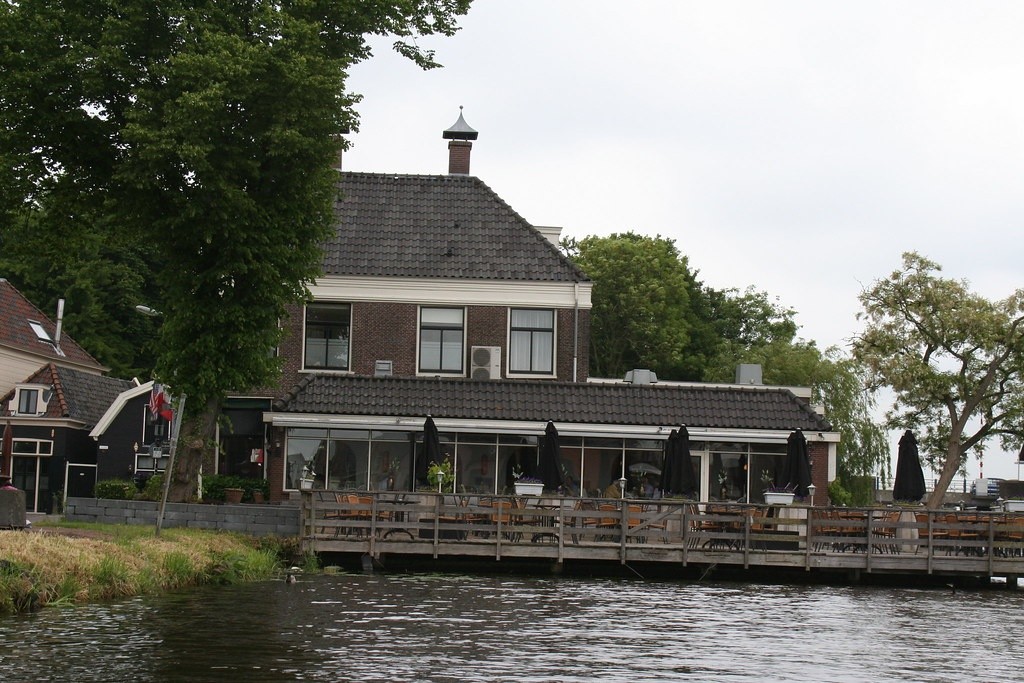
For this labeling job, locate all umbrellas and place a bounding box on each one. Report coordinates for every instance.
[893,430,927,503]
[786,428,812,497]
[544,421,563,493]
[659,424,695,494]
[424,415,442,468]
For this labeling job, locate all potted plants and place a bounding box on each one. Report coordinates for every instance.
[225,476,264,504]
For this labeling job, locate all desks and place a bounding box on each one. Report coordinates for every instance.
[701,510,744,551]
[840,515,890,554]
[530,504,571,544]
[375,500,420,540]
[957,519,1006,558]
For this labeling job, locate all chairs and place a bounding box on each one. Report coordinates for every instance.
[318,489,1024,559]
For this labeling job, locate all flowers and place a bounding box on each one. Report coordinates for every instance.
[762,481,798,493]
[516,476,542,484]
[990,501,996,507]
[388,456,400,475]
[1003,492,1024,500]
[300,459,316,480]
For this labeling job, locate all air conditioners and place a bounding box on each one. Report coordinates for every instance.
[471,345,502,378]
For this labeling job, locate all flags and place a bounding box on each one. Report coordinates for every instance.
[149,383,172,421]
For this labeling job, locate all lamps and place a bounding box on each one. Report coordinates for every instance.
[375,360,392,376]
[997,496,1003,506]
[436,470,444,493]
[807,483,816,506]
[618,477,627,498]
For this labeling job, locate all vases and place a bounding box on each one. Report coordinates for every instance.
[989,506,1005,512]
[300,478,314,489]
[514,482,544,497]
[763,493,795,505]
[1003,500,1024,512]
[387,475,394,490]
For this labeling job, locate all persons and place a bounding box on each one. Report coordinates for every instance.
[643,476,654,495]
[565,476,577,497]
[603,479,622,497]
